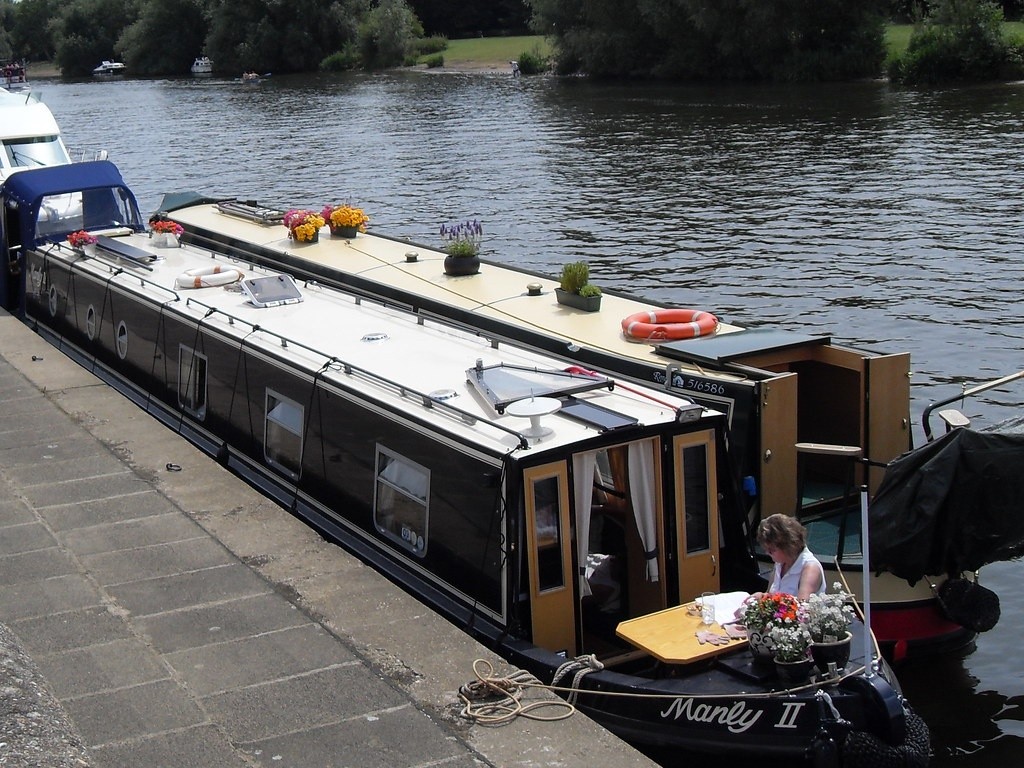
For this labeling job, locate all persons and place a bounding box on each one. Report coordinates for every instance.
[743,513,826,603]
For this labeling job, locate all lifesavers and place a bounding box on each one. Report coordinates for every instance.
[621,309,718,338]
[177,263,241,288]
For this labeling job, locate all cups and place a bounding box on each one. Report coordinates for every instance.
[702,591,715,625]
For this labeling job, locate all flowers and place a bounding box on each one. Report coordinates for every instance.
[283,208,325,241]
[320,203,370,233]
[151,220,183,236]
[67,230,96,249]
[734,582,856,662]
[440,218,483,258]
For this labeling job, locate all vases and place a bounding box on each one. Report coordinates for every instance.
[304,228,319,242]
[444,255,480,275]
[330,223,357,237]
[809,630,852,673]
[746,624,780,662]
[774,657,810,679]
[152,231,180,248]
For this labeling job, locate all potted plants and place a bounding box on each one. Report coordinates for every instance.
[554,260,602,312]
[74,243,95,255]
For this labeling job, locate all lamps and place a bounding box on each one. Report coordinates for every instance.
[676,404,703,424]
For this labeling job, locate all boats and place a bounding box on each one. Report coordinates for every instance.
[190,57,214,78]
[0,63,29,89]
[0,84,109,244]
[92,59,126,74]
[0,160,931,768]
[140,191,1003,674]
[239,72,270,84]
